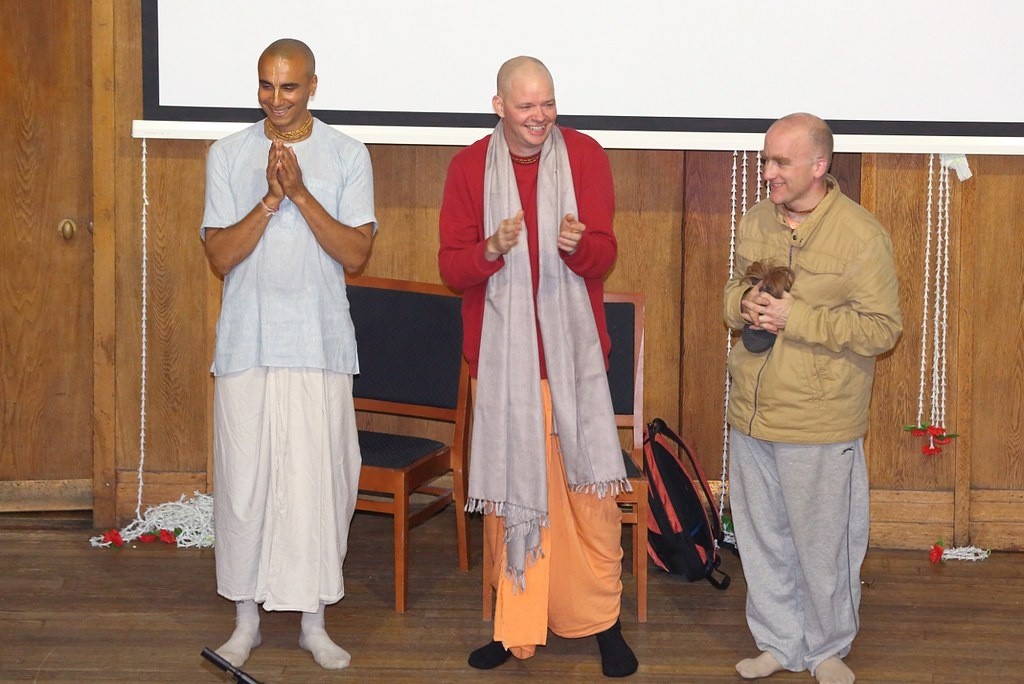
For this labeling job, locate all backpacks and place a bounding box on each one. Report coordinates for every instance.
[641,417,741,591]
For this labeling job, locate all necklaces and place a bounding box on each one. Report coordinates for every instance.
[265,110,315,142]
[511,155,540,165]
[783,191,829,214]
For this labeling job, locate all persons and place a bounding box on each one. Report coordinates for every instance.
[723,111,902,684]
[199,35,378,672]
[439,54,641,678]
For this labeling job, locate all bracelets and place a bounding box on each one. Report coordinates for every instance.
[260,197,280,217]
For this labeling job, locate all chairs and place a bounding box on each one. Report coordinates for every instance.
[346,272,650,623]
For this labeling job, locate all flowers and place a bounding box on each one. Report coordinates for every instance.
[929,540,946,566]
[103,527,183,549]
[903,421,960,456]
